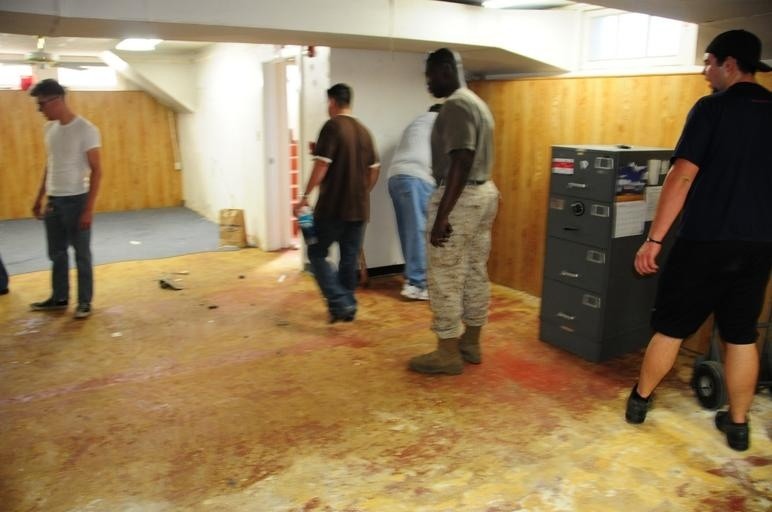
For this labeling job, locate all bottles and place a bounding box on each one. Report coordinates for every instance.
[297,204,318,245]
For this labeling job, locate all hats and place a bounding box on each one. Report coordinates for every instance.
[705,30,772,72]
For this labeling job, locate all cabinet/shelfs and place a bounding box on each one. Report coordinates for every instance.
[538,144,678,364]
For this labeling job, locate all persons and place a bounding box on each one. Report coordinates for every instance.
[410,48,502,374]
[625,30,772,451]
[388,103,442,299]
[29,78,102,319]
[297,84,381,322]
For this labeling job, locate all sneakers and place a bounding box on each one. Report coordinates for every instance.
[403,281,432,302]
[328,301,357,323]
[75,303,90,317]
[410,347,466,375]
[625,394,655,423]
[464,343,484,366]
[30,298,68,310]
[716,410,749,450]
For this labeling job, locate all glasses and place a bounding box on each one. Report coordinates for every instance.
[36,95,61,109]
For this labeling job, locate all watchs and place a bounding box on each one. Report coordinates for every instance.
[644,236,662,245]
[301,192,309,200]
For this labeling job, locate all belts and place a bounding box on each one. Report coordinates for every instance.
[439,177,486,187]
